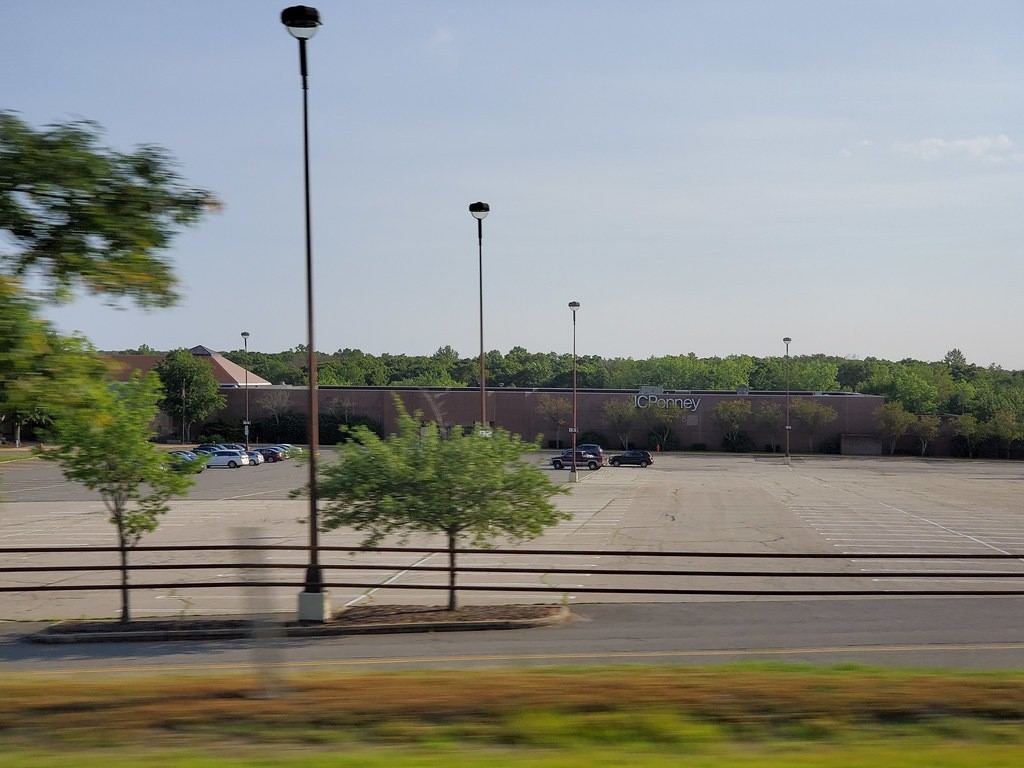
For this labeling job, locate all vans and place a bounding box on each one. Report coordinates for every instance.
[203,449,249,468]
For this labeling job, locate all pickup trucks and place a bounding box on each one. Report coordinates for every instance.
[549,451,607,470]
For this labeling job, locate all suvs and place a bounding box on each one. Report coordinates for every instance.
[562,443,603,456]
[608,450,654,468]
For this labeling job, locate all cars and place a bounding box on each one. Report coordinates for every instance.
[223,444,252,451]
[251,448,281,463]
[271,443,303,461]
[245,451,264,466]
[163,444,227,473]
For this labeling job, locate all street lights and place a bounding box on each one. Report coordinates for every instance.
[469,201,491,437]
[568,300,581,483]
[783,337,793,464]
[241,332,249,445]
[281,4,336,620]
